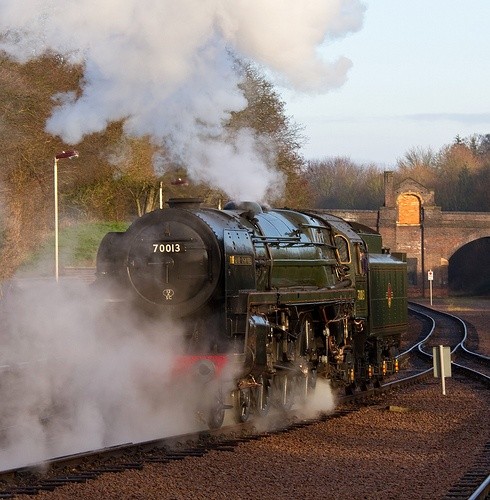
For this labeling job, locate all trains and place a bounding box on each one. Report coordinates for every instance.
[77,193,407,430]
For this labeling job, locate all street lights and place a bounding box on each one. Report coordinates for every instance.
[159,178,190,210]
[54,150,80,286]
